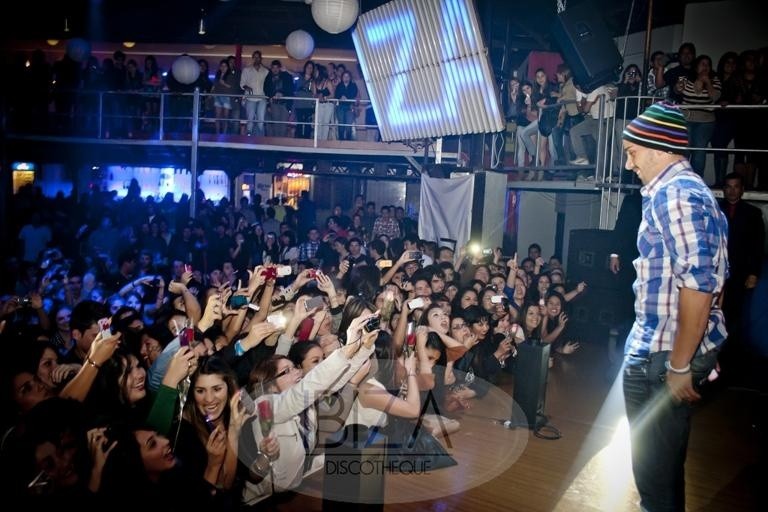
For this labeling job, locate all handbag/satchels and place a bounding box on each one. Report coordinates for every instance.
[387,420,456,472]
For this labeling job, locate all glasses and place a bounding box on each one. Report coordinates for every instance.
[453,322,470,329]
[272,363,302,380]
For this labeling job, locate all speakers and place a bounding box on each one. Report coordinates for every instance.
[322,423,387,512]
[509,339,550,430]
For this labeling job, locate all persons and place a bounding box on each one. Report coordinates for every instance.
[0,37,767,512]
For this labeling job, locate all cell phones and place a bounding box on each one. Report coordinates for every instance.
[184,262,191,272]
[408,251,423,259]
[239,387,255,415]
[258,267,276,279]
[379,259,392,268]
[177,328,192,358]
[186,328,193,342]
[408,297,425,309]
[229,294,247,305]
[491,295,503,303]
[539,298,545,306]
[98,317,111,339]
[276,265,292,276]
[304,295,324,311]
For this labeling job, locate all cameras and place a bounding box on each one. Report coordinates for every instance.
[309,270,323,279]
[362,312,381,333]
[628,71,635,78]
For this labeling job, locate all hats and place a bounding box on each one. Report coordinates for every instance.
[620,101,689,157]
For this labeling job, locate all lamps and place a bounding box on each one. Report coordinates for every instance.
[171,53,203,86]
[286,30,316,61]
[311,0,359,35]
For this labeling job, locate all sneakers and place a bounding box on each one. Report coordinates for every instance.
[569,158,589,166]
